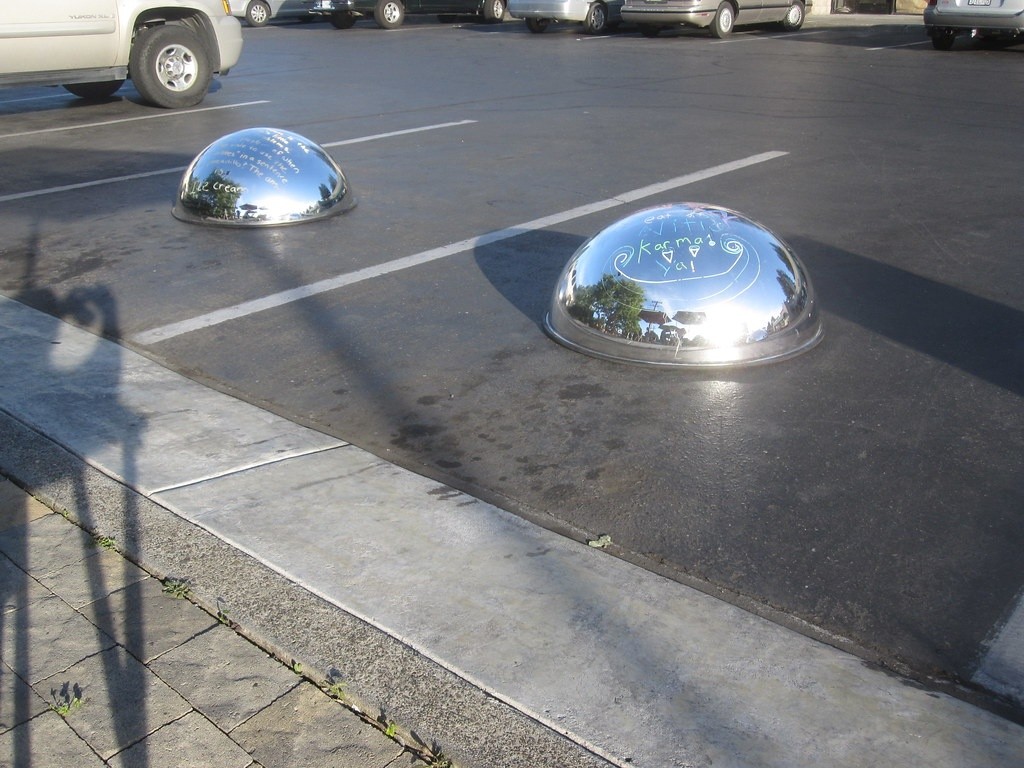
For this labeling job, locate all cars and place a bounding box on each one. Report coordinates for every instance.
[0,0,244,108]
[923,0,1024,52]
[620,0,814,40]
[505,0,625,34]
[309,0,507,28]
[222,0,316,27]
